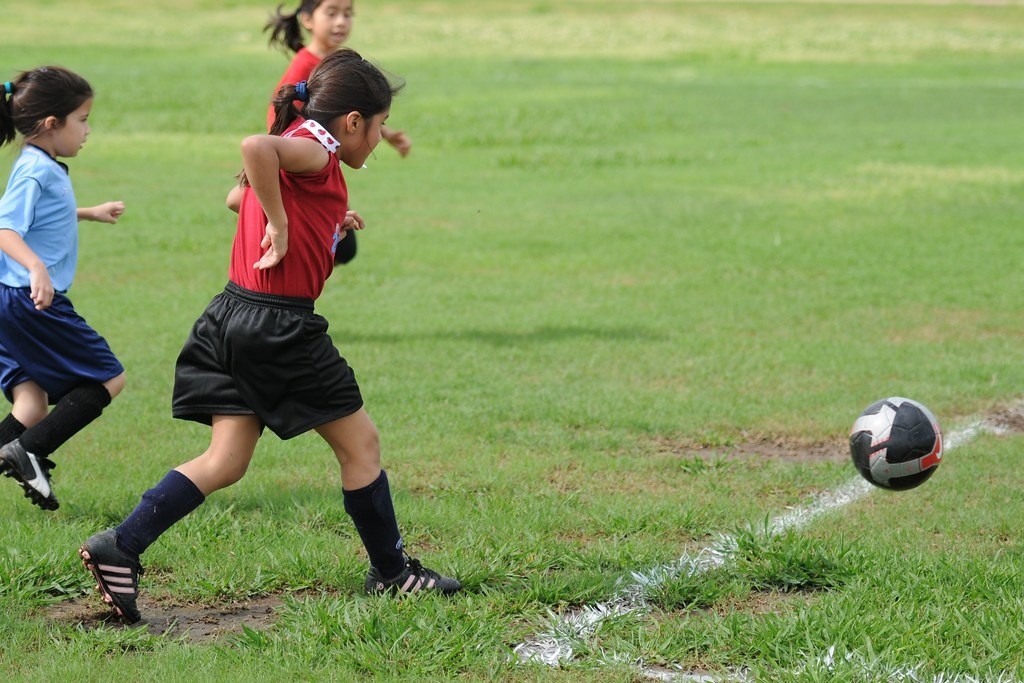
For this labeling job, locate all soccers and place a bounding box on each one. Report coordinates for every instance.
[847,397,944,492]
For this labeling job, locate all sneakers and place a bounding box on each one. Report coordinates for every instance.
[78,527,145,625]
[363,557,463,598]
[0,438,60,512]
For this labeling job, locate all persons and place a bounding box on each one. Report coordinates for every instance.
[0,65,127,511]
[265,0,412,265]
[77,49,463,625]
